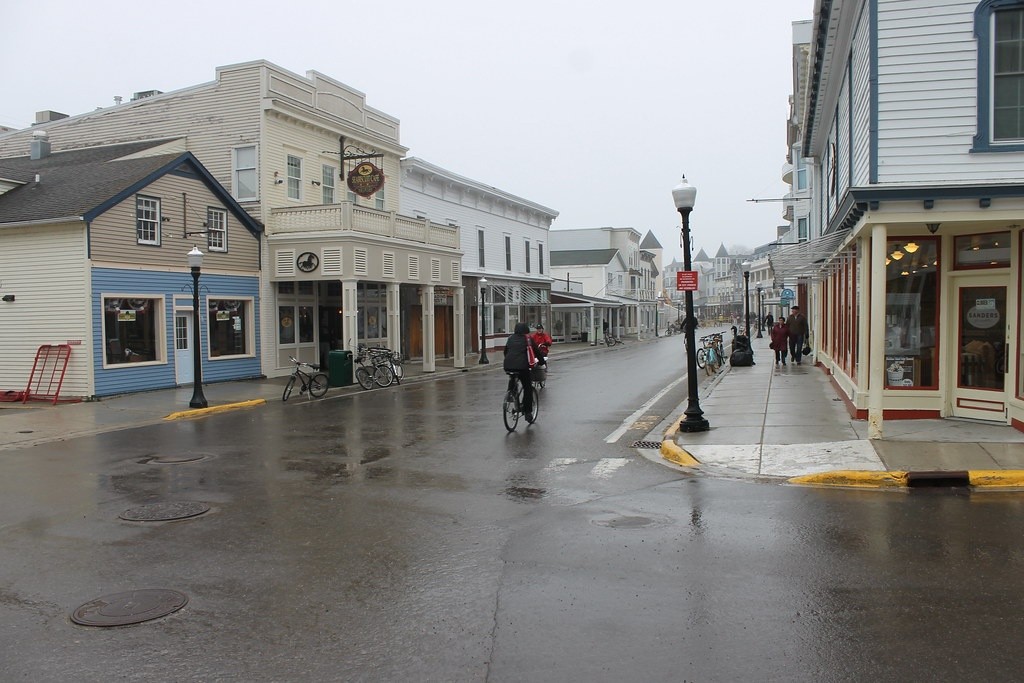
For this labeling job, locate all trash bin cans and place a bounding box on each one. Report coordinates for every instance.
[581,331,588,343]
[326,349,353,387]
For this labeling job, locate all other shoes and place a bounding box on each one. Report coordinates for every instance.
[782,360,786,366]
[791,356,794,362]
[776,360,778,364]
[524,414,534,421]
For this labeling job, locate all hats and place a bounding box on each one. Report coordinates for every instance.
[535,324,544,329]
[791,306,799,309]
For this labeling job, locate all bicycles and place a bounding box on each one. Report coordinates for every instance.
[604,331,624,347]
[681,328,688,352]
[503,360,547,432]
[533,342,549,388]
[665,326,683,336]
[697,330,727,375]
[346,337,405,390]
[282,355,329,401]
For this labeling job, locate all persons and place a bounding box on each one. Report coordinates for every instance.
[504,322,546,421]
[786,306,809,364]
[771,317,797,365]
[680,317,698,344]
[698,313,737,327]
[531,324,552,356]
[765,312,773,335]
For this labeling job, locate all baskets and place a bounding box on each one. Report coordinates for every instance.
[532,367,547,381]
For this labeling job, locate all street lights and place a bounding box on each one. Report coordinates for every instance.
[479,275,489,364]
[677,300,680,323]
[654,296,658,336]
[180,244,211,408]
[741,260,751,339]
[761,291,765,331]
[756,281,763,338]
[672,175,710,433]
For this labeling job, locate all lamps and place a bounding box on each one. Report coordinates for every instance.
[162,217,170,221]
[274,171,283,184]
[903,241,920,253]
[312,181,321,186]
[926,224,940,234]
[890,244,904,261]
[2,295,15,301]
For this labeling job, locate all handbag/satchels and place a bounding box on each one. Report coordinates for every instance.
[527,335,537,367]
[802,345,811,355]
[769,341,774,349]
